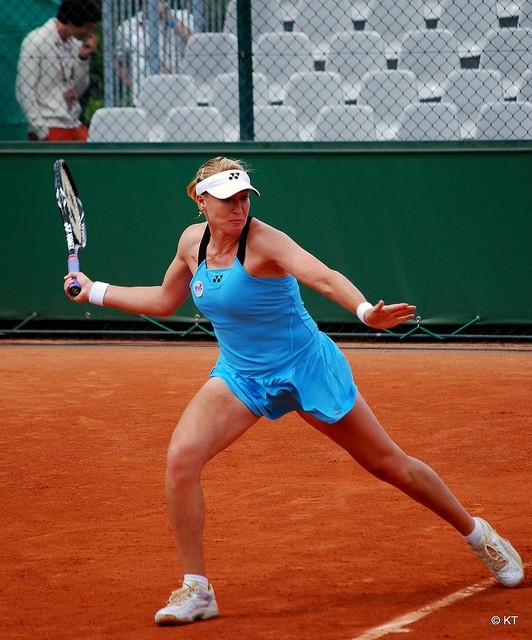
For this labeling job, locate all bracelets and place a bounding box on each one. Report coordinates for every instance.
[89,280,110,307]
[356,301,374,326]
[170,17,177,28]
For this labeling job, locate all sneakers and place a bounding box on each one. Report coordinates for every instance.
[155,582,219,625]
[464,517,525,588]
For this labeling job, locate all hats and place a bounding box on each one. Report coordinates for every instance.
[195,169,261,200]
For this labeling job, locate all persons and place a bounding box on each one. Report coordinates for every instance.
[15,0,102,141]
[115,0,194,107]
[63,154,526,627]
